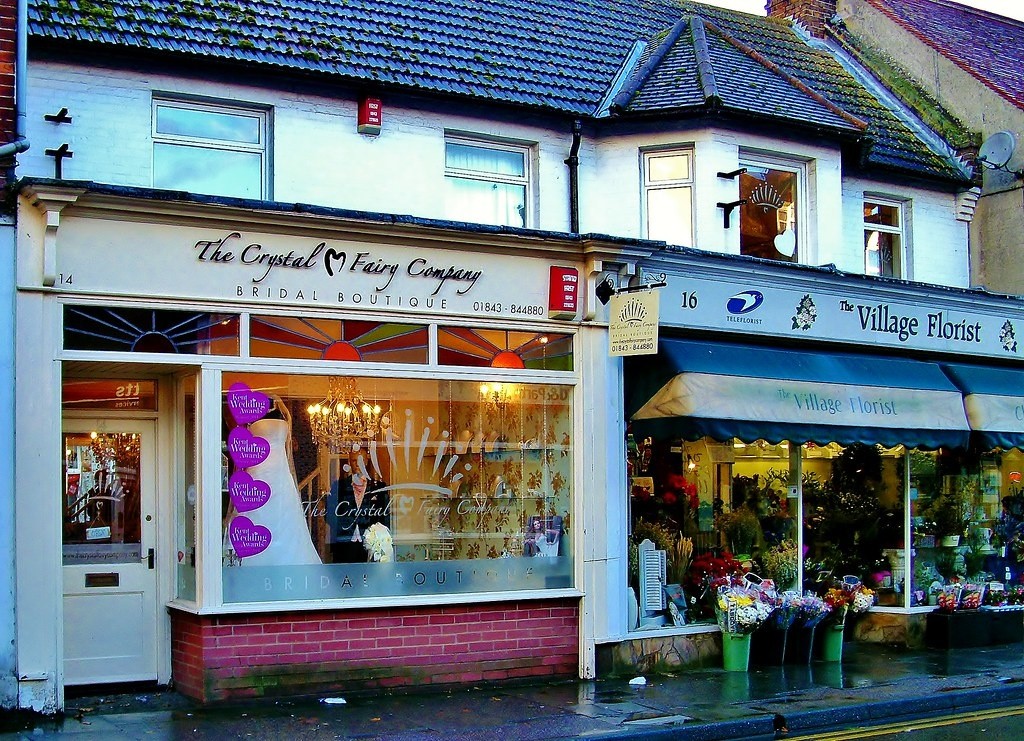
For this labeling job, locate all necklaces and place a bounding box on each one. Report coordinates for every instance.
[456,433,503,497]
[267,407,275,413]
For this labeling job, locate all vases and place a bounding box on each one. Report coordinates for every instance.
[926,610,1024,648]
[722,624,844,670]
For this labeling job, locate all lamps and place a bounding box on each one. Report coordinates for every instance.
[307,374,390,444]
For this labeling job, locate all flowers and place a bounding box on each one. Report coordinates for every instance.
[628,444,876,632]
[883,485,1024,612]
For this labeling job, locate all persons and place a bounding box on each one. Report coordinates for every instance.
[87,470,113,522]
[222,396,324,566]
[523,519,559,558]
[324,447,391,564]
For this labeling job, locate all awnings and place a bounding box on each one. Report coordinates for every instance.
[622,338,1024,454]
